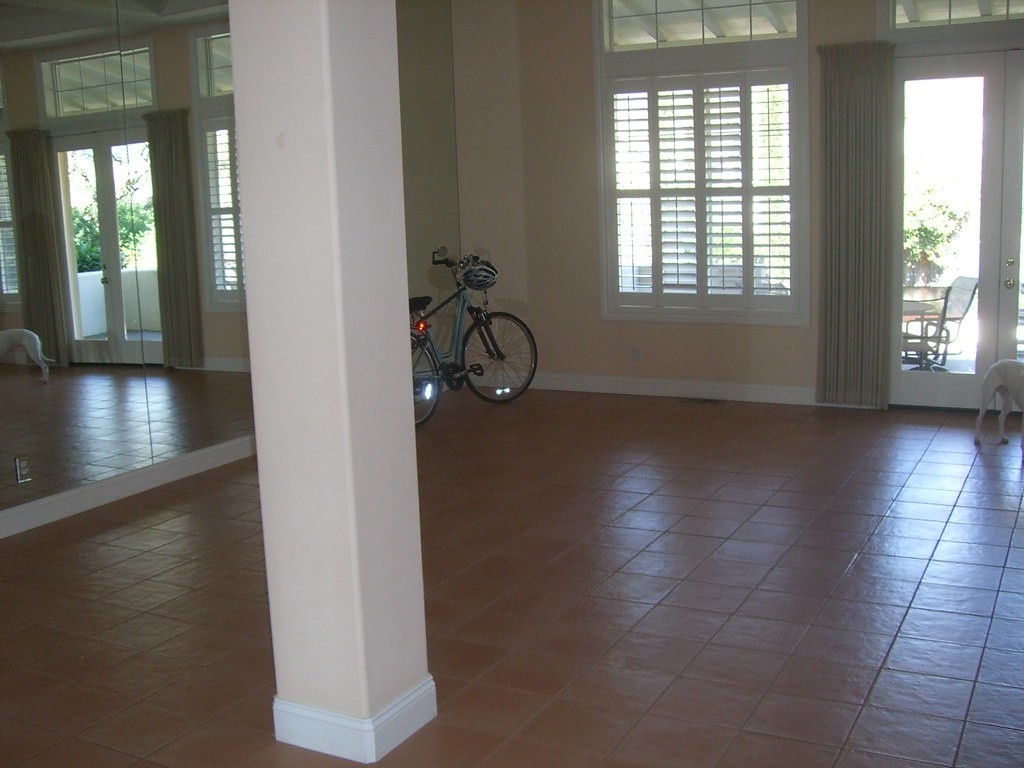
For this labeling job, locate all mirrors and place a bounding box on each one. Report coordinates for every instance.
[1,0,260,514]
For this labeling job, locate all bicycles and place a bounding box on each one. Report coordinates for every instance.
[409,246,538,426]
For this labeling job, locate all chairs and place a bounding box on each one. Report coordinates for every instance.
[902,276,982,373]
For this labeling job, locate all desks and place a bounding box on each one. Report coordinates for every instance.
[902,301,935,364]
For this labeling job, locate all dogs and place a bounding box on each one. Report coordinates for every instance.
[0,329,56,385]
[974,359,1024,446]
